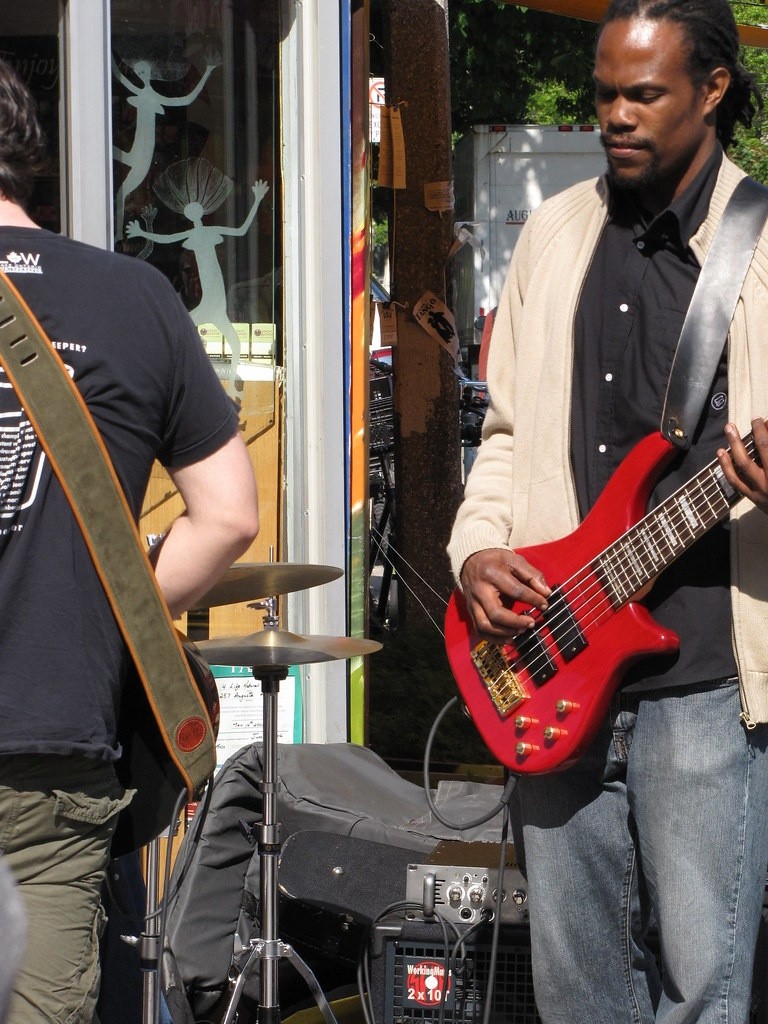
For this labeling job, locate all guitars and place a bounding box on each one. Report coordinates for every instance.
[443,412,767,783]
[112,628,217,855]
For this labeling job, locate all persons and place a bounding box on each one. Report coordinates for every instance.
[0,61,259,1024]
[448,0,768,1024]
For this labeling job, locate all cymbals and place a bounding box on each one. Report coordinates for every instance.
[152,562,349,612]
[185,631,386,671]
